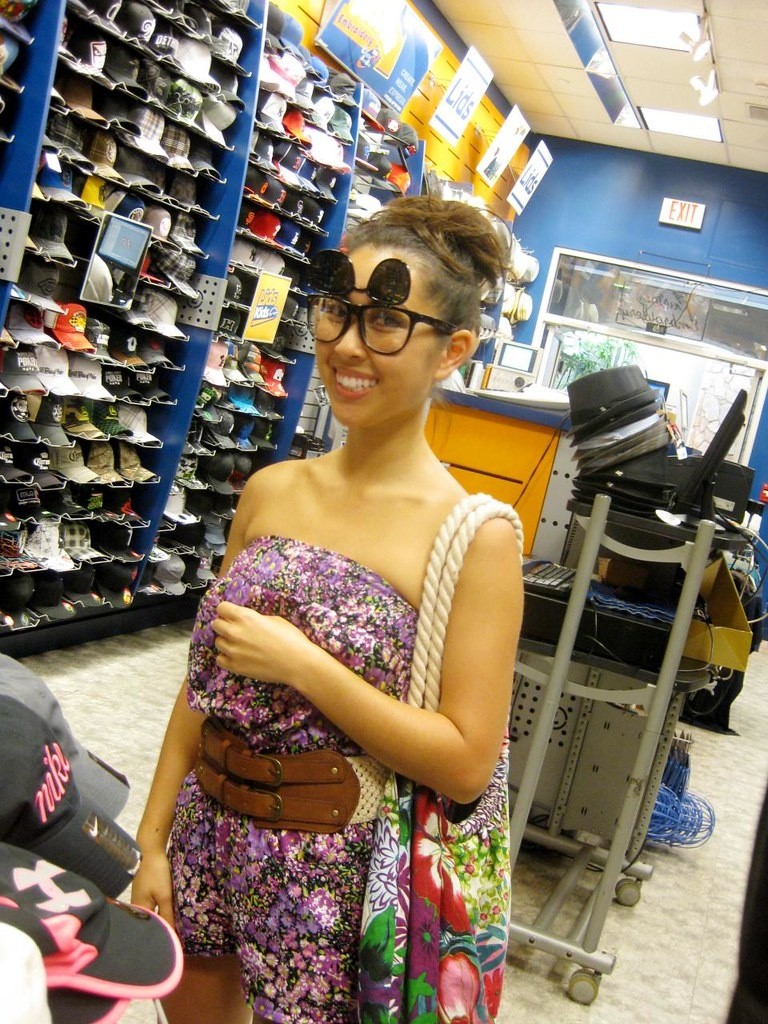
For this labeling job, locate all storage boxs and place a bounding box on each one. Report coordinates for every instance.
[682,550,753,673]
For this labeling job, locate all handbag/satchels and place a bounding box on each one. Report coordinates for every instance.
[359,491,511,1024]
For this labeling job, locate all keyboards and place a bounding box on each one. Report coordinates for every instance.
[522,560,579,596]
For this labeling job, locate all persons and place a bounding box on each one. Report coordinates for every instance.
[132,197,524,1024]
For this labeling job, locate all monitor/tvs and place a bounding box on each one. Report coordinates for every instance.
[669,389,747,532]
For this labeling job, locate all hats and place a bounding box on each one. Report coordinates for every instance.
[0,694,144,900]
[1,648,131,817]
[565,362,681,518]
[0,841,184,1024]
[0,0,539,633]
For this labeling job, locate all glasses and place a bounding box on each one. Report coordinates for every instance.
[304,249,458,356]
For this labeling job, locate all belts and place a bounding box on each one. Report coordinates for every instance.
[195,716,387,832]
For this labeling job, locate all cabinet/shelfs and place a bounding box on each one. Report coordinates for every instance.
[1,0,510,656]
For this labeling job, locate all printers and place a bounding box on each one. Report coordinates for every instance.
[666,453,755,525]
